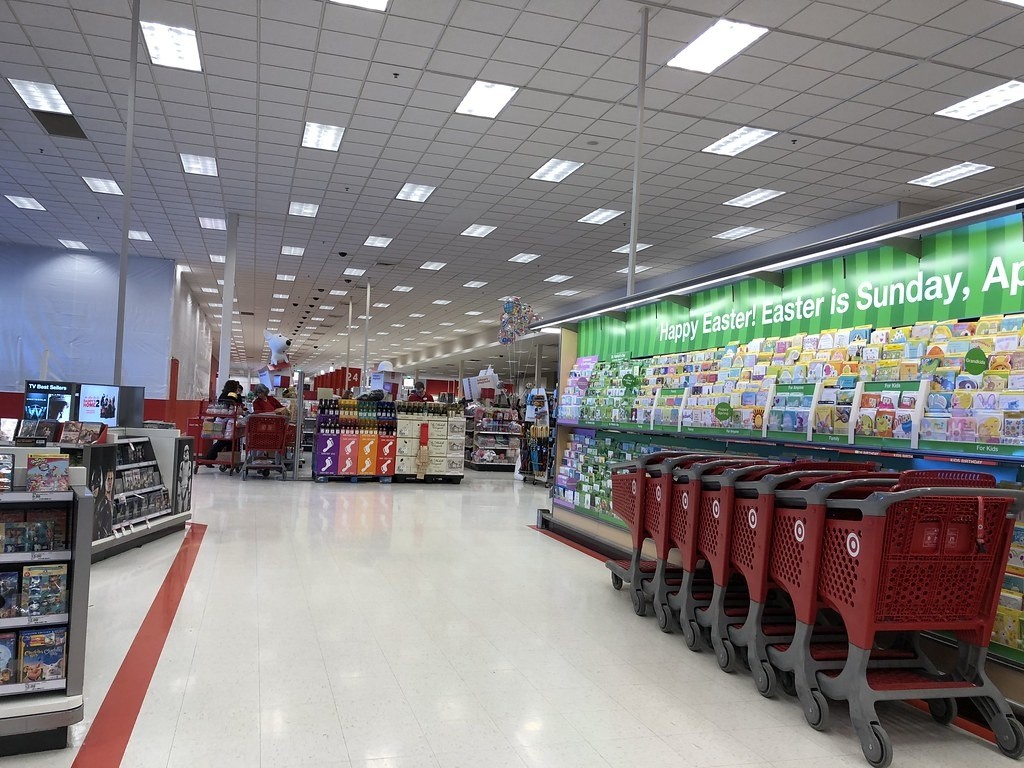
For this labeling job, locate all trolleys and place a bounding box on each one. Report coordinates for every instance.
[605,449,1024,767]
[192,398,246,476]
[284,422,305,471]
[241,413,288,481]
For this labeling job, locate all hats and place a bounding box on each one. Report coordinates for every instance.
[184,445,189,451]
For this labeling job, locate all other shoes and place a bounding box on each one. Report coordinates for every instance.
[256,469,263,477]
[205,464,214,468]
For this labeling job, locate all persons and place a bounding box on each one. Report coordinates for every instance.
[205,380,252,468]
[341,390,354,399]
[39,426,52,441]
[102,397,115,418]
[177,445,192,512]
[282,386,292,398]
[68,424,76,432]
[49,395,70,421]
[249,384,286,414]
[82,430,97,443]
[90,465,113,542]
[64,435,69,443]
[237,385,244,402]
[408,382,434,402]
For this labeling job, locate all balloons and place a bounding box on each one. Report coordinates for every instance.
[504,296,522,317]
[496,303,543,347]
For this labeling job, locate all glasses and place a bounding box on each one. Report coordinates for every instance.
[256,391,264,395]
[417,390,423,393]
